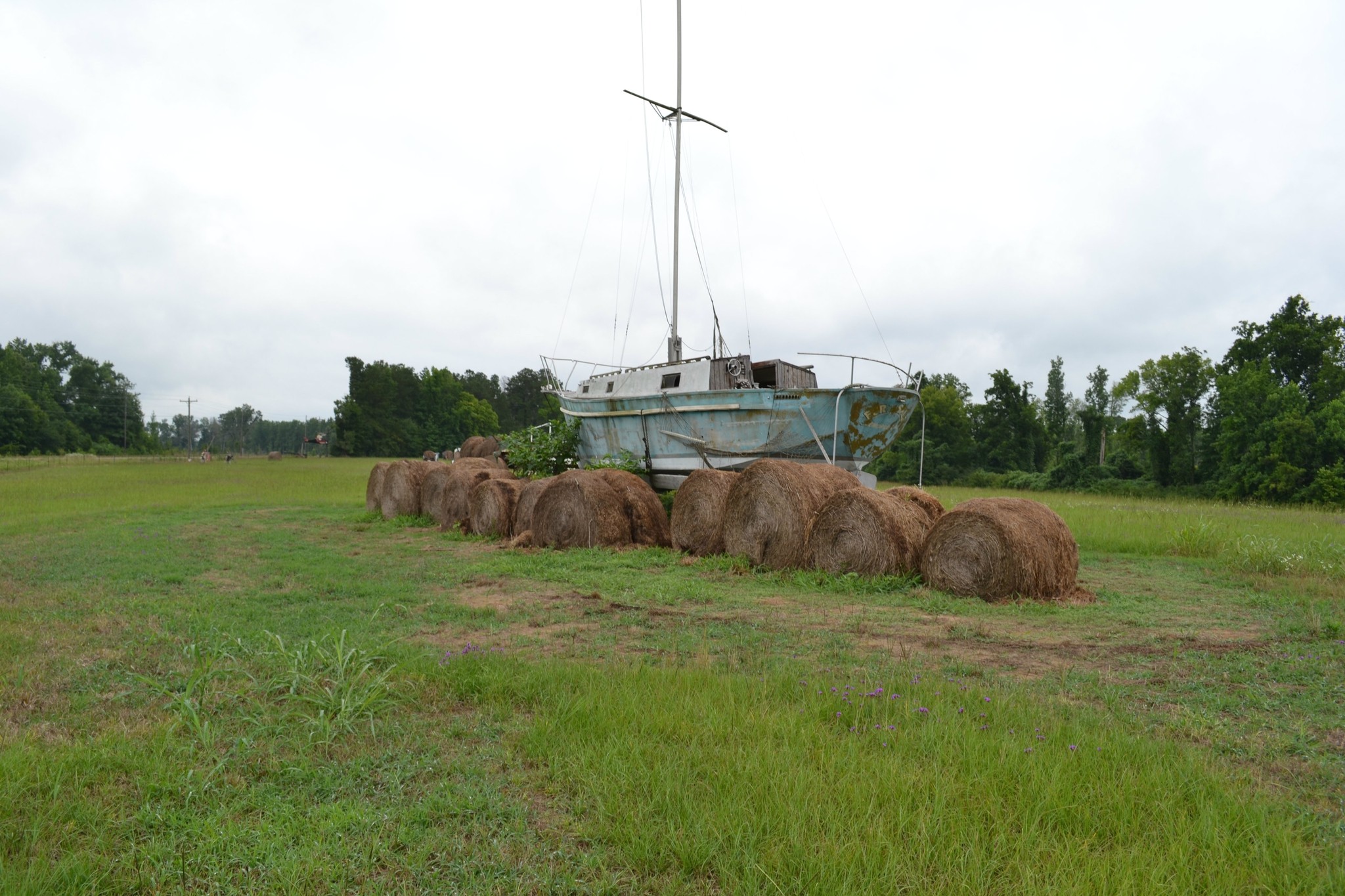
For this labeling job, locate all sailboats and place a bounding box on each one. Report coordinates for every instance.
[527,0,928,493]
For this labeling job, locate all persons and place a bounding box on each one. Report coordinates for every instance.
[201,449,210,463]
[223,453,235,465]
